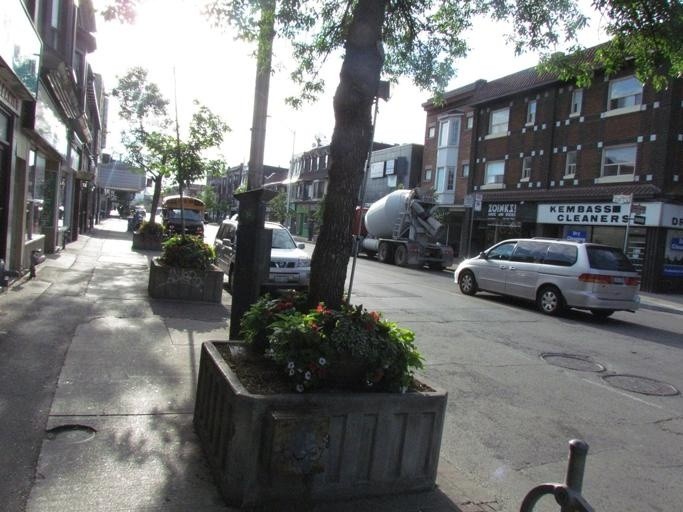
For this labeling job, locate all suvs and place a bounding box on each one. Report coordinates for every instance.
[211,214,311,294]
[136,208,145,217]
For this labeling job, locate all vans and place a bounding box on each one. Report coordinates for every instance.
[452,237,640,319]
[134,206,144,212]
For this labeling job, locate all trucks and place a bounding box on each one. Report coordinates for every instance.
[160,194,209,242]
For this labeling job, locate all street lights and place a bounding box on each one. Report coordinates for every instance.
[265,113,296,227]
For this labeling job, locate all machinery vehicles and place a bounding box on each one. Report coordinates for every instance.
[349,188,454,270]
